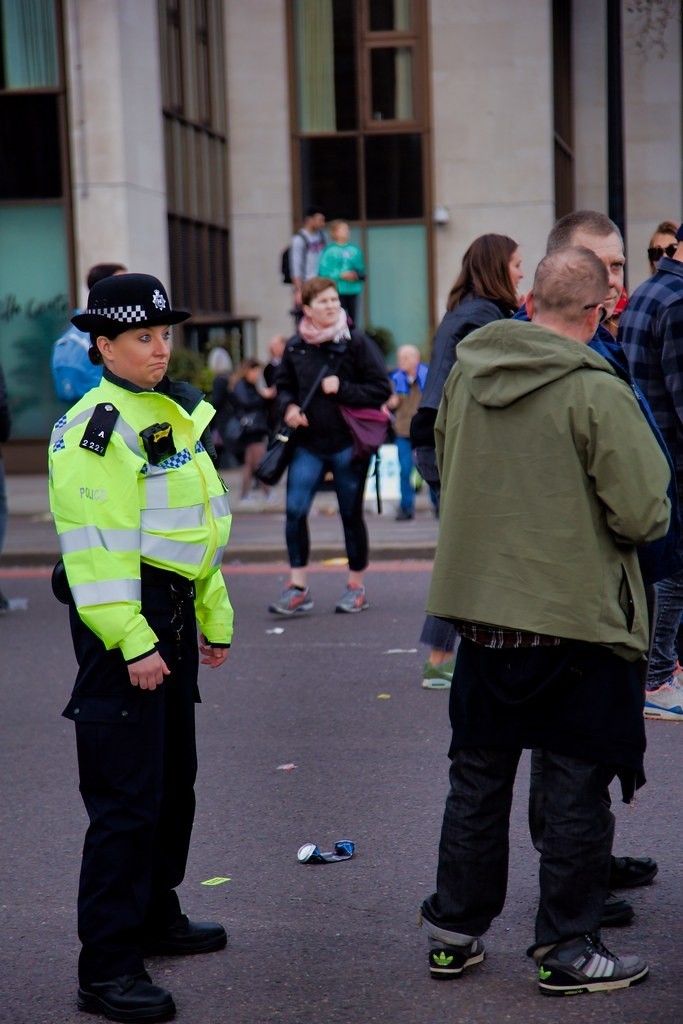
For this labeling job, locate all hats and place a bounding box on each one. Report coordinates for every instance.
[71,272,191,332]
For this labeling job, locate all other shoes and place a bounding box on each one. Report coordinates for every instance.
[396,513,413,521]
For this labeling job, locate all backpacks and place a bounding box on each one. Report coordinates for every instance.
[281,230,325,284]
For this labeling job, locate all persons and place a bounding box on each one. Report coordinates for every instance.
[647,221,679,275]
[51,264,127,401]
[509,209,683,928]
[378,344,441,522]
[418,244,672,997]
[409,234,525,690]
[615,223,683,721]
[208,209,367,504]
[268,277,393,615]
[48,273,235,1024]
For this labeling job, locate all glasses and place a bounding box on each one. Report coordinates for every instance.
[647,244,678,261]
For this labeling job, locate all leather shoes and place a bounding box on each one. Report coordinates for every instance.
[140,919,227,954]
[77,978,175,1018]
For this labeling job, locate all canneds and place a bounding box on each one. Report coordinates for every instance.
[297,840,354,863]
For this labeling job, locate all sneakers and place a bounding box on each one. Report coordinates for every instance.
[422,654,456,688]
[268,582,312,614]
[643,677,683,720]
[428,937,485,974]
[537,932,649,994]
[669,659,683,683]
[334,582,368,613]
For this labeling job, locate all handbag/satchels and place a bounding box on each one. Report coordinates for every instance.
[255,433,293,485]
[341,408,389,458]
